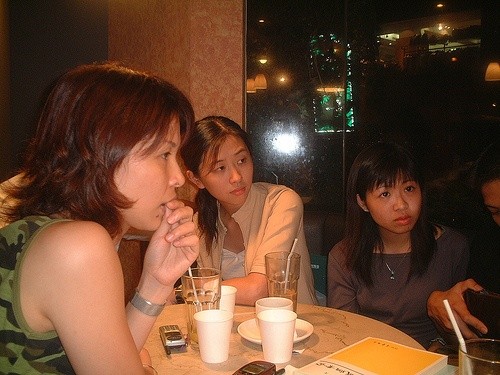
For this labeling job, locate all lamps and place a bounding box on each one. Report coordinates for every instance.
[484,61,500,82]
[253,73,267,89]
[247,78,256,93]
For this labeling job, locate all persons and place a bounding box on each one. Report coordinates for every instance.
[326,142,470,351]
[167,116,319,306]
[426,151,500,341]
[0,64,199,375]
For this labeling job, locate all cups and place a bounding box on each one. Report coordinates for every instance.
[265,251,301,312]
[458,338,500,375]
[193,310,234,363]
[181,267,222,350]
[257,310,297,363]
[220,285,237,313]
[255,297,294,316]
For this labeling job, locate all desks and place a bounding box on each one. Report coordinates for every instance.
[142,304,428,375]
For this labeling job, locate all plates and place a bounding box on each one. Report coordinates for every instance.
[237,317,314,344]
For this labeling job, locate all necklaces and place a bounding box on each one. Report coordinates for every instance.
[383,247,410,280]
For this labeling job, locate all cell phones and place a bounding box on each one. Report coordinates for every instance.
[159,325,185,355]
[464,289,500,341]
[232,361,286,375]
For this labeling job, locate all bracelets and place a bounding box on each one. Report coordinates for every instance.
[130,289,166,316]
[175,286,185,304]
